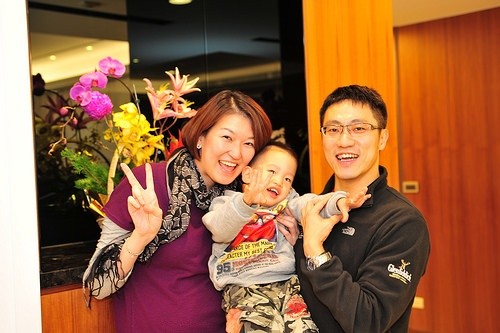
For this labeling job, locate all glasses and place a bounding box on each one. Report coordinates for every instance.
[320,123,382,138]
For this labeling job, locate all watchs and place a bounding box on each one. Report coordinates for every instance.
[306,251,332,271]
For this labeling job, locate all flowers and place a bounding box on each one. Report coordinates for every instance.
[31,73,111,171]
[69,59,202,218]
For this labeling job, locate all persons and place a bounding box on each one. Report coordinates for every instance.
[83,90,272,333]
[225,84,431,333]
[201,139,371,332]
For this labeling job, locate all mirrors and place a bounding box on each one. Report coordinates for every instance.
[25,0,291,271]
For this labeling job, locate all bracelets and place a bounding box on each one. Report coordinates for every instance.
[125,237,145,256]
